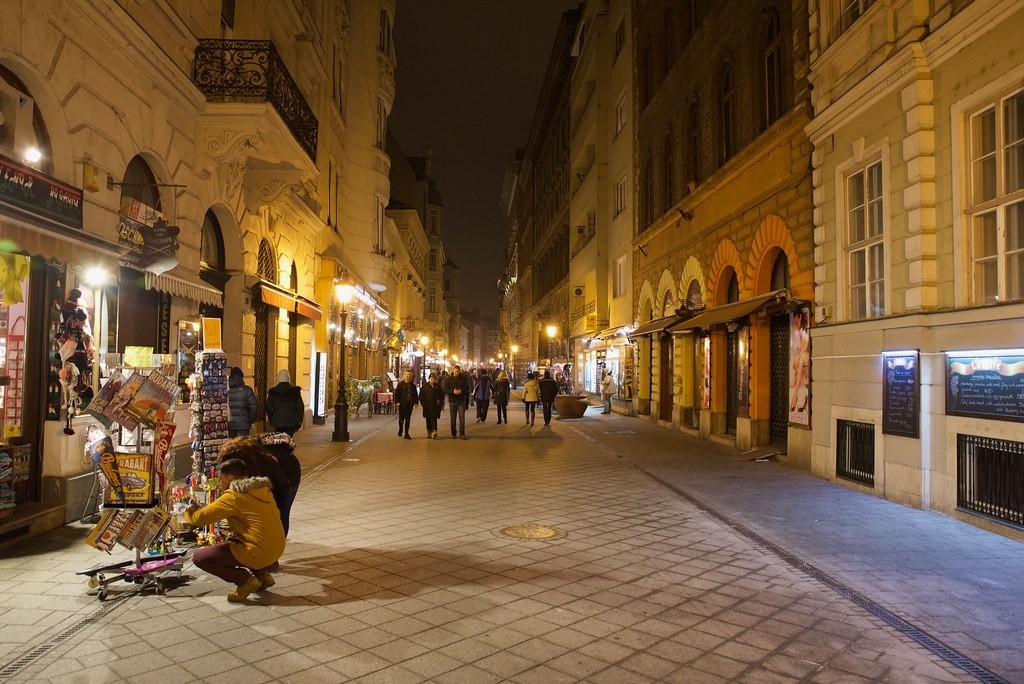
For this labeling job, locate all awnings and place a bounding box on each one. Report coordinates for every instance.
[629,314,683,336]
[666,288,787,331]
[254,285,322,322]
[0,209,122,283]
[122,254,224,309]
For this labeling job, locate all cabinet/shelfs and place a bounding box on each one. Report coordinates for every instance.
[73,363,187,602]
[189,349,228,491]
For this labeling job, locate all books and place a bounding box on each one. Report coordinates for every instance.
[84,369,183,505]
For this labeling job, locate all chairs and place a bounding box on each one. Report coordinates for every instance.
[373,391,396,414]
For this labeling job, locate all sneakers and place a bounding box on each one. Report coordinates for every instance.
[227,575,261,603]
[251,569,275,593]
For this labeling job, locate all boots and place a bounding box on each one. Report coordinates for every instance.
[525,411,529,424]
[531,412,535,425]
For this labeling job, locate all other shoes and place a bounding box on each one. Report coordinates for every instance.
[547,419,550,424]
[460,435,467,440]
[398,429,403,437]
[267,562,279,571]
[480,420,486,424]
[432,433,438,439]
[427,434,432,438]
[601,411,610,414]
[497,420,502,425]
[450,431,456,439]
[476,417,481,423]
[544,423,547,426]
[504,416,507,424]
[404,434,412,439]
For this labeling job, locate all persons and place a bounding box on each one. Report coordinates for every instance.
[445,366,469,440]
[183,432,301,603]
[419,373,445,439]
[266,369,305,438]
[601,368,616,414]
[436,367,513,410]
[790,311,810,411]
[225,367,258,439]
[473,370,494,424]
[395,370,419,439]
[522,371,575,425]
[493,371,511,424]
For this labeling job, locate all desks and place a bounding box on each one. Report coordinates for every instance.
[378,392,394,403]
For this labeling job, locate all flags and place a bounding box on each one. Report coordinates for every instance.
[396,327,406,342]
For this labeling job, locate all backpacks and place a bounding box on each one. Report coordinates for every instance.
[249,434,297,471]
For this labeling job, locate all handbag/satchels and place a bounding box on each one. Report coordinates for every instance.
[436,409,441,418]
[537,399,540,406]
[494,392,500,400]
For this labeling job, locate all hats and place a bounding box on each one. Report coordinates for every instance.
[278,369,290,382]
[544,371,550,376]
[231,367,244,378]
[481,370,487,375]
[429,374,436,379]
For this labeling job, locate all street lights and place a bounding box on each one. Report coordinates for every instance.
[498,351,502,369]
[331,274,352,443]
[420,335,429,388]
[511,343,519,391]
[442,347,447,372]
[546,320,558,369]
